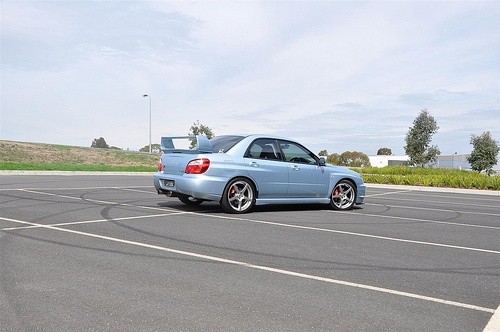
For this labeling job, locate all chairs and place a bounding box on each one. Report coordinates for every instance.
[259,145,278,159]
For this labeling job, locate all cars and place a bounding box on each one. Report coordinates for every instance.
[153,133,365,214]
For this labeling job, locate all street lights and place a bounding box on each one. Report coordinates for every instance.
[143,93,152,154]
